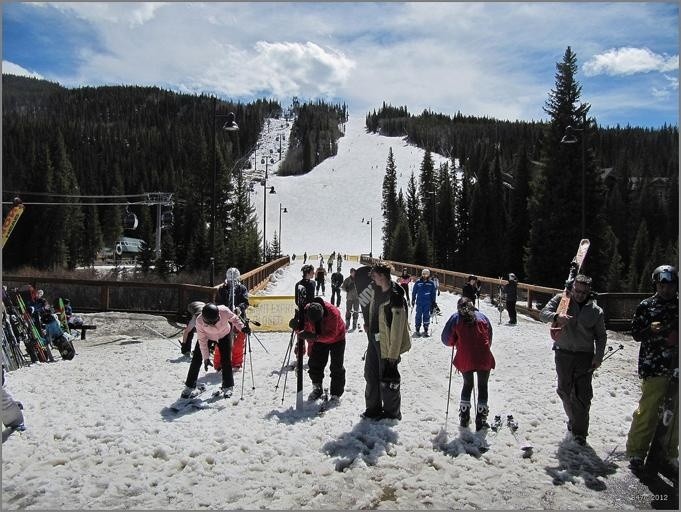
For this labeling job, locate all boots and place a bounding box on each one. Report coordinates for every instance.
[476,404,489,424]
[459,400,471,422]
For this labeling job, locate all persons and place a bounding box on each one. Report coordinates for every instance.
[291,250,520,368]
[0,363,27,433]
[302,296,348,402]
[211,267,251,372]
[626,263,680,470]
[182,301,208,362]
[440,295,495,433]
[63,296,73,323]
[540,274,608,453]
[179,299,252,398]
[361,261,415,422]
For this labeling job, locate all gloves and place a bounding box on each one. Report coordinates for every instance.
[242,327,251,335]
[299,331,317,339]
[289,319,299,331]
[204,359,213,371]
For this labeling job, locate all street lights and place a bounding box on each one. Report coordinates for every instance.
[559,126,587,271]
[208,111,240,288]
[426,182,437,267]
[264,179,277,265]
[366,217,373,257]
[279,203,288,259]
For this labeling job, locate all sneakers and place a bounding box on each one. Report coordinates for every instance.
[329,395,339,405]
[309,389,323,399]
[630,455,644,469]
[567,422,585,446]
[224,387,232,397]
[363,408,381,419]
[376,410,401,421]
[182,385,195,395]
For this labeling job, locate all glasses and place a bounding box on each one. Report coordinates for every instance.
[575,287,588,295]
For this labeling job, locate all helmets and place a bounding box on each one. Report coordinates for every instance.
[650,265,678,286]
[226,267,240,286]
[202,302,219,323]
